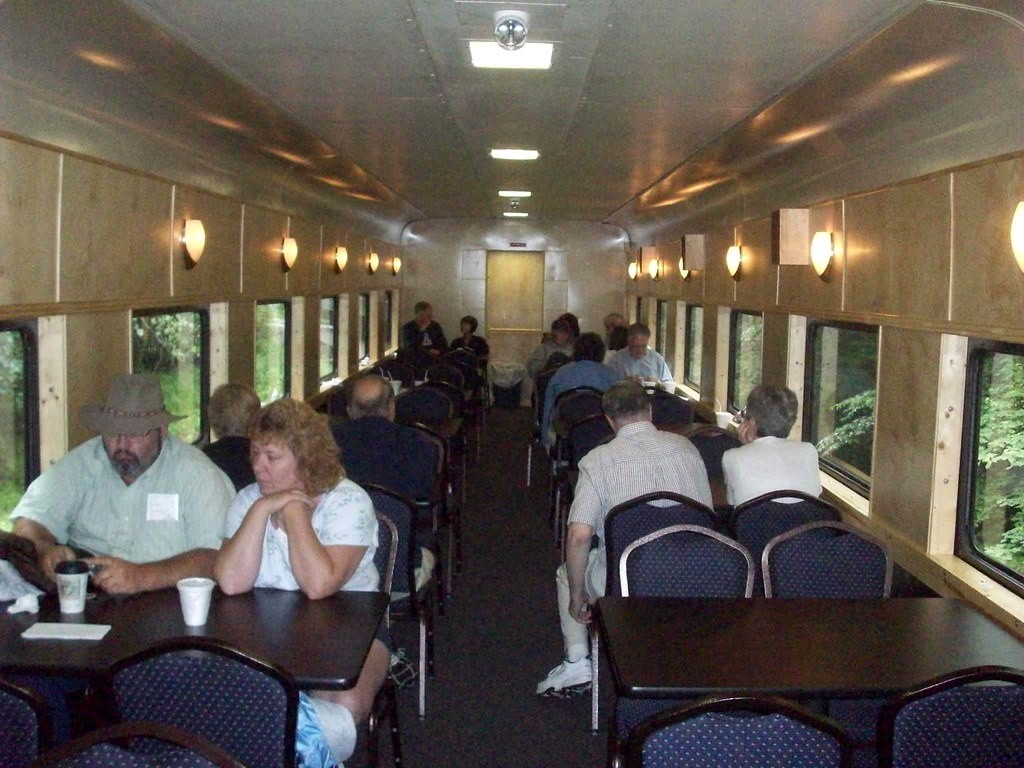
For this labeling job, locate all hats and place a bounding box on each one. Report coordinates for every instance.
[77,373,189,437]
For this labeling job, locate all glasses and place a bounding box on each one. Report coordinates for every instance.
[102,430,153,444]
[741,410,748,417]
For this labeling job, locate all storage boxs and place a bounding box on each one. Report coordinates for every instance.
[492,361,525,409]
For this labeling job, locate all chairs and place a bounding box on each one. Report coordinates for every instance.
[0,511,401,768]
[329,349,489,720]
[527,369,745,562]
[587,491,1024,768]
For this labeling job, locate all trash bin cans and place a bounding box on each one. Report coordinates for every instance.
[490,362,524,409]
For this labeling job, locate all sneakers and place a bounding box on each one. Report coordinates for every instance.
[537,655,593,700]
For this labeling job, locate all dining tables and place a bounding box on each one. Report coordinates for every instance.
[709,479,727,506]
[331,415,464,437]
[0,586,391,690]
[595,597,1024,717]
[554,418,723,439]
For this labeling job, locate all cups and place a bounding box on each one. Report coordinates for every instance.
[383,377,389,384]
[389,381,402,397]
[176,578,217,626]
[414,380,427,387]
[54,562,89,615]
[644,382,656,394]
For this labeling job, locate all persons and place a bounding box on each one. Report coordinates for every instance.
[450,316,490,354]
[536,379,714,701]
[213,398,389,726]
[721,383,824,506]
[9,374,235,594]
[202,383,261,489]
[329,375,435,602]
[403,302,448,355]
[526,314,673,468]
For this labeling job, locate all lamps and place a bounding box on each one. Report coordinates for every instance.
[183,219,205,268]
[811,232,832,276]
[393,257,401,275]
[678,257,689,279]
[628,263,637,279]
[334,247,349,273]
[726,247,741,277]
[1011,202,1024,273]
[368,253,380,275]
[281,238,299,271]
[649,260,658,279]
